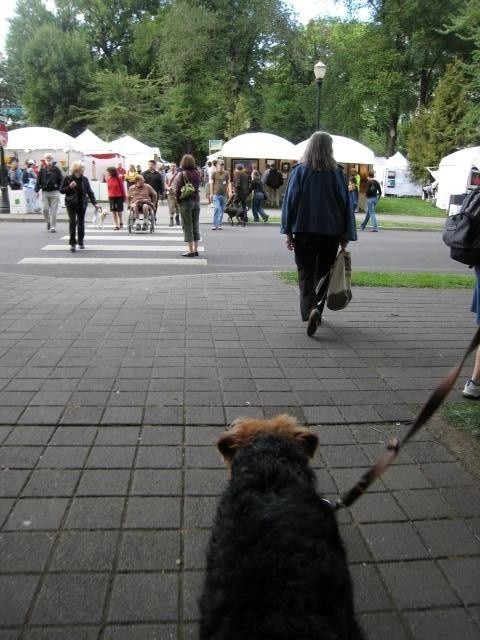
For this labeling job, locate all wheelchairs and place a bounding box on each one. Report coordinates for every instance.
[126,201,157,234]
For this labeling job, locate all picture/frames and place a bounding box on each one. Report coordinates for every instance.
[230,158,290,184]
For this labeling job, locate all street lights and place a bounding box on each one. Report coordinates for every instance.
[313,60,329,129]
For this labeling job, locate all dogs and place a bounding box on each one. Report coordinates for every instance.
[97,209,108,230]
[224,206,247,226]
[196,415,364,640]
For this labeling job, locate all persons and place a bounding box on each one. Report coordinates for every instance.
[358,164,369,194]
[0,152,283,233]
[360,171,382,233]
[59,160,102,252]
[348,176,359,210]
[280,130,358,335]
[174,152,201,257]
[460,266,479,400]
[350,167,361,213]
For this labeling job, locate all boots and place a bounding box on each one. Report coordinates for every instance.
[169,217,173,226]
[175,215,180,225]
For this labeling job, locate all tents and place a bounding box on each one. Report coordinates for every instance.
[374,149,424,198]
[435,144,480,216]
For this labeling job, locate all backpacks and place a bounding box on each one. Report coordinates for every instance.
[442,185,480,268]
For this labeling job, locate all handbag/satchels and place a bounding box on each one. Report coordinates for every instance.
[370,182,375,191]
[179,184,196,201]
[166,188,172,195]
[325,248,352,310]
[254,191,265,200]
[65,193,79,207]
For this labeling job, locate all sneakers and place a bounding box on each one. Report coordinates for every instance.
[70,247,76,252]
[142,223,148,230]
[135,223,141,230]
[47,222,50,230]
[462,379,480,399]
[212,226,216,229]
[114,227,121,230]
[49,227,56,232]
[181,252,195,257]
[217,225,222,229]
[78,243,84,248]
[306,309,321,336]
[120,223,124,228]
